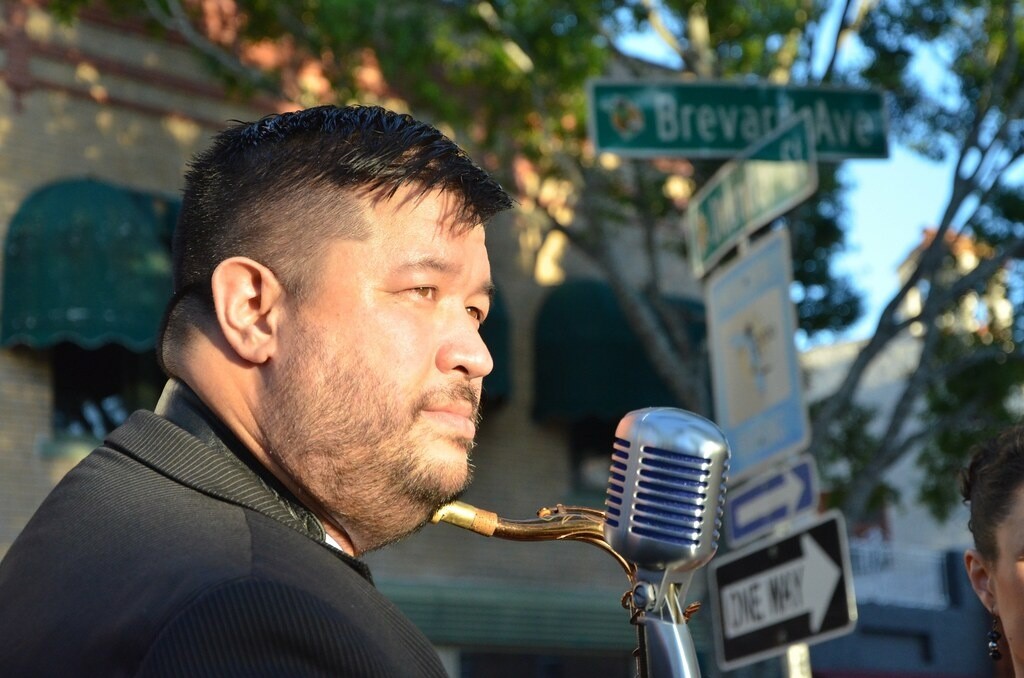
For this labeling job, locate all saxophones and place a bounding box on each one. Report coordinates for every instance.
[427,479,708,678]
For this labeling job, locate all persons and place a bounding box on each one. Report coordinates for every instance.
[964,426,1024,677]
[0,104,513,678]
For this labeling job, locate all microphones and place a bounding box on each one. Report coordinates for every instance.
[602,407,732,677]
